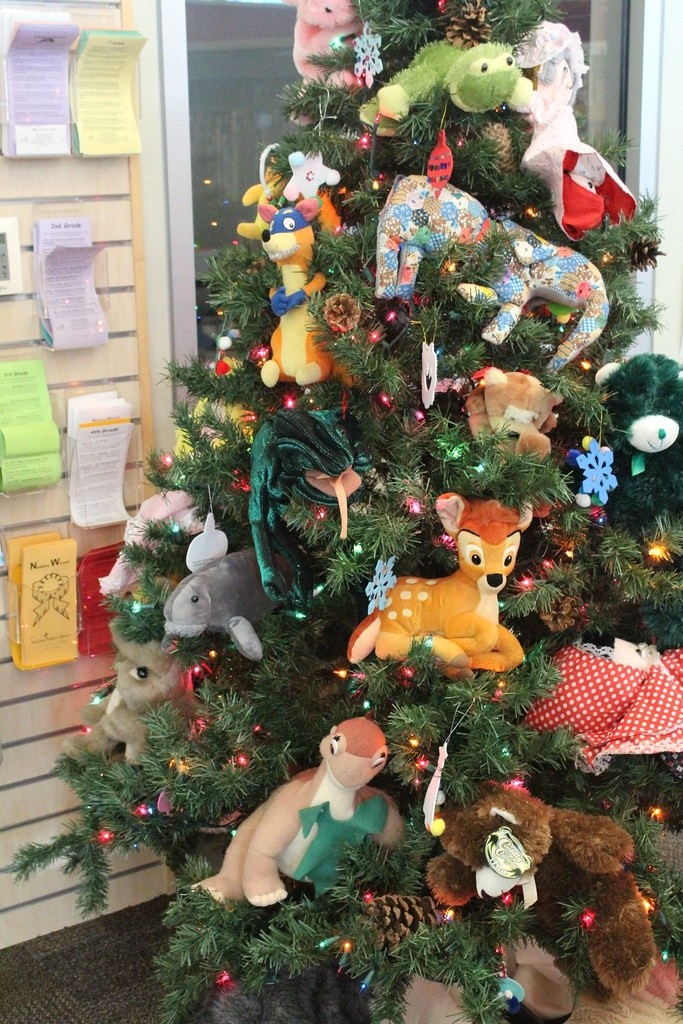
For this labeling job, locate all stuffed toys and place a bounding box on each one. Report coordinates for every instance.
[59,1,683,1024]
